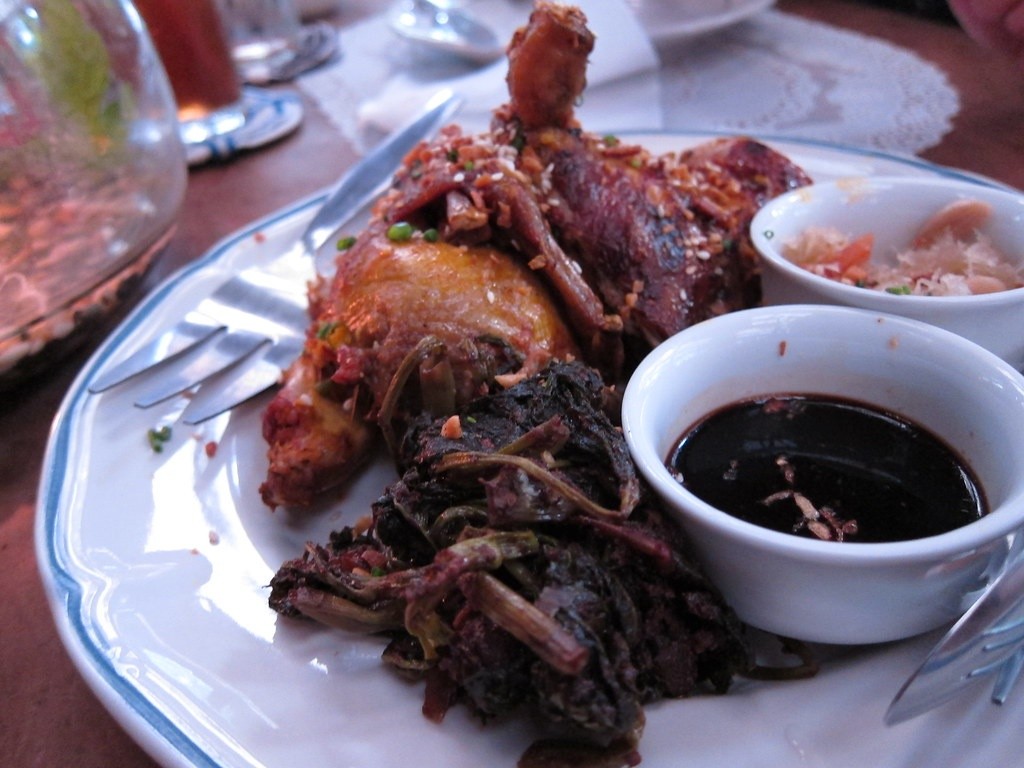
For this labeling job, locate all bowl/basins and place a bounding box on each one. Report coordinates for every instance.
[750,175,1024,371]
[620,305,1023,645]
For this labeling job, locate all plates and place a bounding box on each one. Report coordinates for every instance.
[265,16,337,84]
[387,0,776,68]
[187,85,303,165]
[31,122,1024,768]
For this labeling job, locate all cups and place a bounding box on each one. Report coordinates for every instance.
[213,1,300,85]
[133,0,244,142]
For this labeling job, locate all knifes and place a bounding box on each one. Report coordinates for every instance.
[881,551,1022,728]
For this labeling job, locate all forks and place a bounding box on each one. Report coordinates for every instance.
[968,620,1024,674]
[89,89,466,425]
